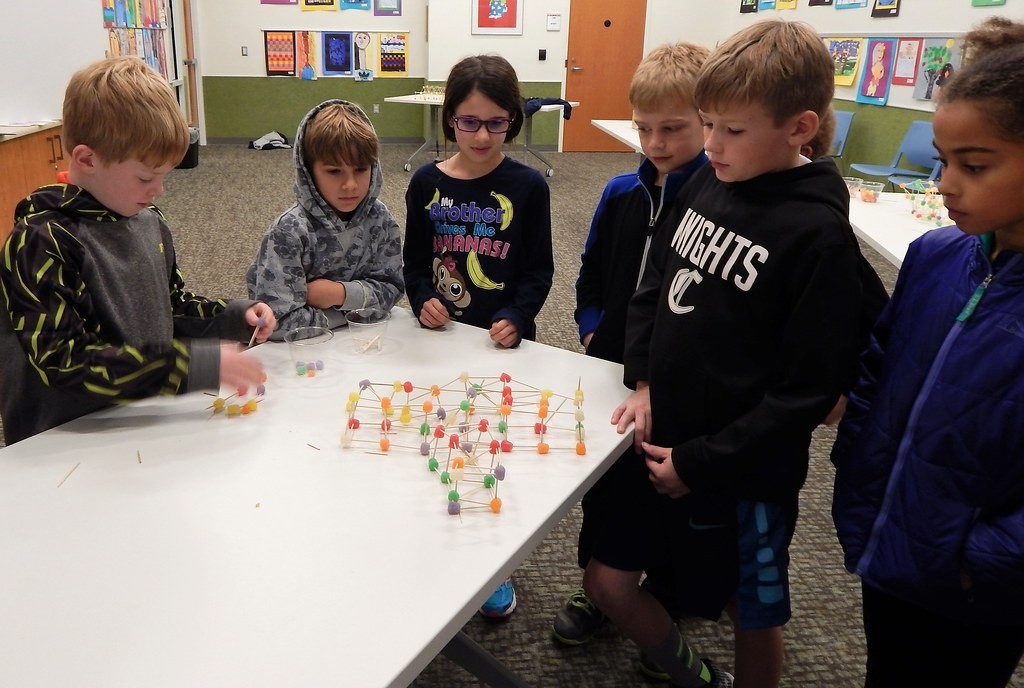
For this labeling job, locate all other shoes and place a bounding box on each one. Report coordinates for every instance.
[640,656,737,688]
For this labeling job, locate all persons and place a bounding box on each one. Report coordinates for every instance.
[553,43,726,650]
[828,16,1023,687]
[610,20,891,686]
[403,58,553,615]
[0,54,280,448]
[242,95,405,342]
[801,108,835,164]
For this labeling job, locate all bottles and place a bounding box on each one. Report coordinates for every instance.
[420,85,447,103]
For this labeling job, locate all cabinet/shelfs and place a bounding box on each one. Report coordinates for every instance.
[0,125,70,253]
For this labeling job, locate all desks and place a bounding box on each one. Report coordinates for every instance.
[850,193,957,271]
[384,92,580,178]
[591,119,645,157]
[0,305,635,687]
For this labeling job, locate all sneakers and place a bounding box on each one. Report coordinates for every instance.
[552,580,603,645]
[479,582,516,615]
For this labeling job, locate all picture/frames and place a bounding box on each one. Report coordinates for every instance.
[471,0,524,36]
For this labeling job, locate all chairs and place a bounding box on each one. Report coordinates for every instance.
[847,120,940,194]
[832,110,854,179]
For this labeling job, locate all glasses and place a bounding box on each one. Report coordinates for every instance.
[450,112,514,134]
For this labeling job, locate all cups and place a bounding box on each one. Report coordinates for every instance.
[842,176,863,199]
[861,181,885,203]
[284,326,334,378]
[345,308,392,354]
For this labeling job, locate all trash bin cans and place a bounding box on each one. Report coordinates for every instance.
[176,127,200,169]
